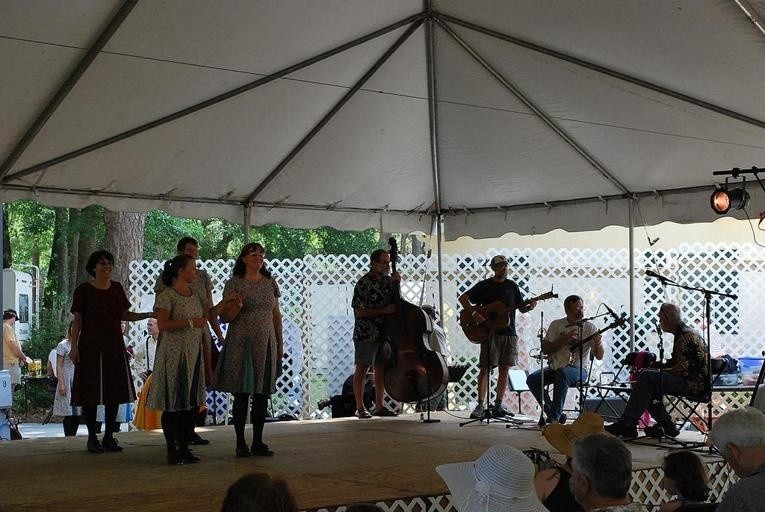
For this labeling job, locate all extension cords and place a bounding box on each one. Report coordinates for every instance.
[532,455,555,475]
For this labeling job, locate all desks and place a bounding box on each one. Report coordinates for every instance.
[21,374,51,418]
[712,384,758,392]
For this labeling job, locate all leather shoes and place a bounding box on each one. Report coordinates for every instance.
[251,443,274,456]
[167,453,184,465]
[87,440,104,452]
[102,438,123,451]
[178,449,201,463]
[236,447,251,458]
[194,436,209,444]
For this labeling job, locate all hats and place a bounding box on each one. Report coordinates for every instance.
[491,255,508,268]
[4,310,19,321]
[544,411,616,459]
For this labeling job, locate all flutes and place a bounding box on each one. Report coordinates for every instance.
[570,318,627,353]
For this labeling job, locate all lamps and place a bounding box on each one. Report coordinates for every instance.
[709,166,765,215]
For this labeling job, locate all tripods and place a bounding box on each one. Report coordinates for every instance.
[460,332,523,427]
[619,349,687,447]
[668,296,727,462]
[506,311,553,435]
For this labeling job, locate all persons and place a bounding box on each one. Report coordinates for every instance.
[220,472,298,512]
[526,295,604,423]
[459,255,537,418]
[603,303,712,439]
[350,250,402,419]
[710,406,765,512]
[46,236,284,465]
[658,450,709,512]
[568,432,645,512]
[2,308,26,390]
[534,412,615,512]
[415,305,449,413]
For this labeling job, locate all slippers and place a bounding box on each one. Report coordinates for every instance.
[354,408,372,418]
[373,406,399,416]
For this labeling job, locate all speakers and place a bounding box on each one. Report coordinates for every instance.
[582,397,628,425]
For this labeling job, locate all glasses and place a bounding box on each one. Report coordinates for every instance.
[248,252,265,258]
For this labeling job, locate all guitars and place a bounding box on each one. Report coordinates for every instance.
[460,278,557,343]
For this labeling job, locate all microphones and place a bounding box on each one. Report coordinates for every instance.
[604,304,619,319]
[657,325,662,337]
[534,355,547,359]
[646,270,666,280]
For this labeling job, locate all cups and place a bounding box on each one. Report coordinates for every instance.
[34,359,42,376]
[28,363,36,377]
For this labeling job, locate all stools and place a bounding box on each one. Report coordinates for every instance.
[562,383,590,419]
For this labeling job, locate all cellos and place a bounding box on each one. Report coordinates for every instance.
[384,237,450,404]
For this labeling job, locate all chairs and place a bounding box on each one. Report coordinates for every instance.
[651,356,730,443]
[592,350,659,421]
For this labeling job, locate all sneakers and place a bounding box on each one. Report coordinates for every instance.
[470,405,485,419]
[644,423,680,438]
[493,406,515,417]
[604,421,638,438]
